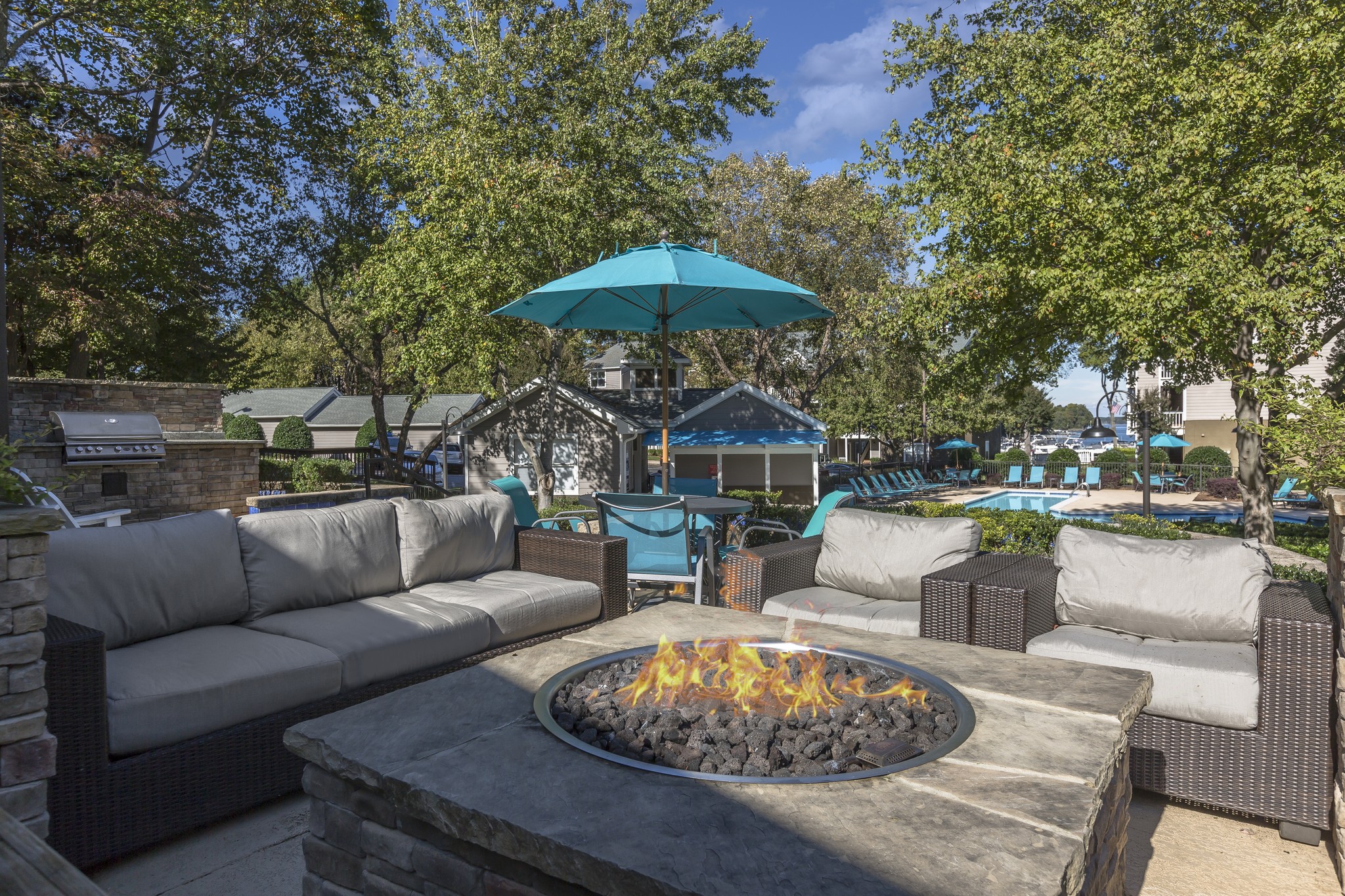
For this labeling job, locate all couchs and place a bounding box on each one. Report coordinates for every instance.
[726,508,1026,643]
[49,492,629,868]
[969,525,1334,847]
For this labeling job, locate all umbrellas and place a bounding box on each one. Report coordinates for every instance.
[487,231,837,492]
[934,437,980,469]
[1135,432,1191,476]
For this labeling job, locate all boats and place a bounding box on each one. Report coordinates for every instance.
[1000,428,1086,455]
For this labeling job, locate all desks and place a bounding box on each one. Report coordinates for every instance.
[1160,474,1178,494]
[946,471,960,487]
[670,495,752,547]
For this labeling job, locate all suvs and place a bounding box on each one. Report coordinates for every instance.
[369,432,414,470]
[433,441,465,472]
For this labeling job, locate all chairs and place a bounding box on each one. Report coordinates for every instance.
[934,468,982,489]
[848,469,952,505]
[10,467,131,528]
[718,490,855,564]
[592,492,715,607]
[653,476,718,549]
[486,476,598,533]
[1273,477,1318,511]
[999,465,1193,494]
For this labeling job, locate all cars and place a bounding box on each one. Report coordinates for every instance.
[392,450,444,488]
[818,462,870,480]
